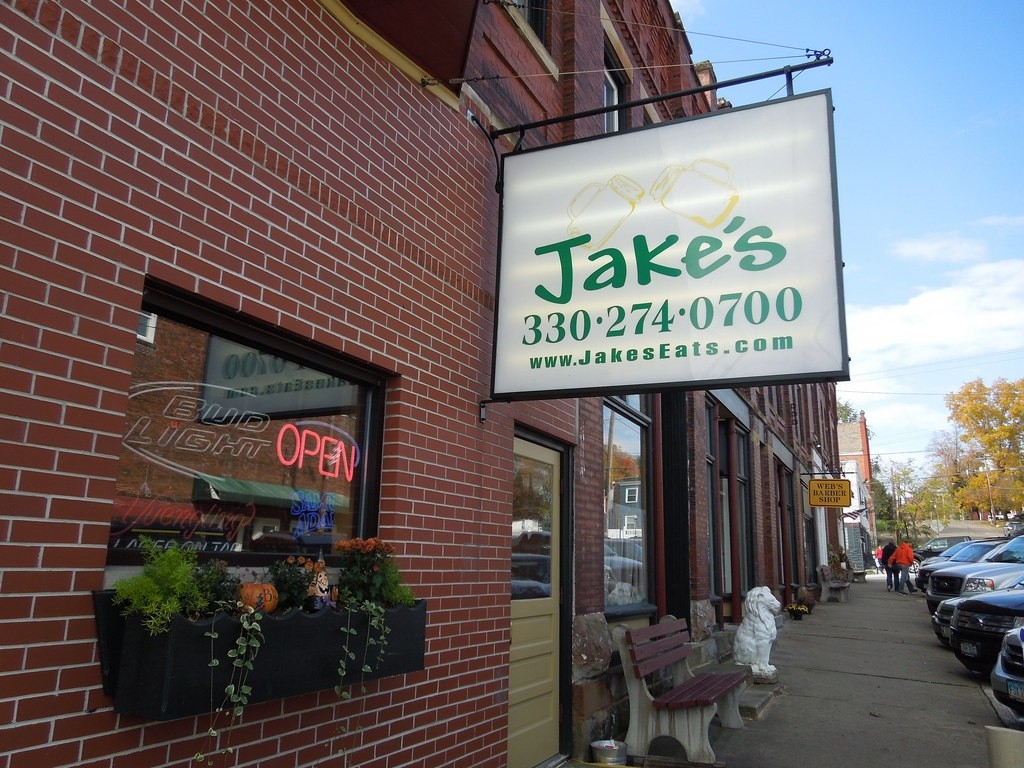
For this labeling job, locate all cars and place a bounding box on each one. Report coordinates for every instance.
[915,536,1015,590]
[990,624,1024,709]
[915,540,1012,594]
[948,573,1024,674]
[931,576,1024,648]
[908,535,972,573]
[1004,521,1024,538]
[511,529,643,608]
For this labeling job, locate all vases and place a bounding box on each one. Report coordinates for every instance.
[794,614,802,620]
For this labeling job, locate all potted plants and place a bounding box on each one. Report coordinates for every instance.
[114,537,429,723]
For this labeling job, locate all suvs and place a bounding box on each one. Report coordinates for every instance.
[925,534,1024,616]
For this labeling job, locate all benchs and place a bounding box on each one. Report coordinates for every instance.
[816,565,851,602]
[612,615,749,768]
[847,562,867,583]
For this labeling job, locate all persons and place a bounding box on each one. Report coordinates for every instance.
[876,545,883,570]
[888,541,918,596]
[881,540,900,592]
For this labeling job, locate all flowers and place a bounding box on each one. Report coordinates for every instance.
[786,604,809,615]
[331,537,396,598]
[269,557,323,607]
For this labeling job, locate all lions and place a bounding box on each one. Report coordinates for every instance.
[732,585,783,676]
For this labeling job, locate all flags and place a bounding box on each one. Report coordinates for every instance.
[839,506,870,519]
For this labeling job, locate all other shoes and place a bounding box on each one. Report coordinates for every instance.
[899,592,907,596]
[887,586,891,591]
[911,589,918,594]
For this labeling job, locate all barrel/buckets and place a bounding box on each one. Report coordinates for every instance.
[590,740,628,765]
